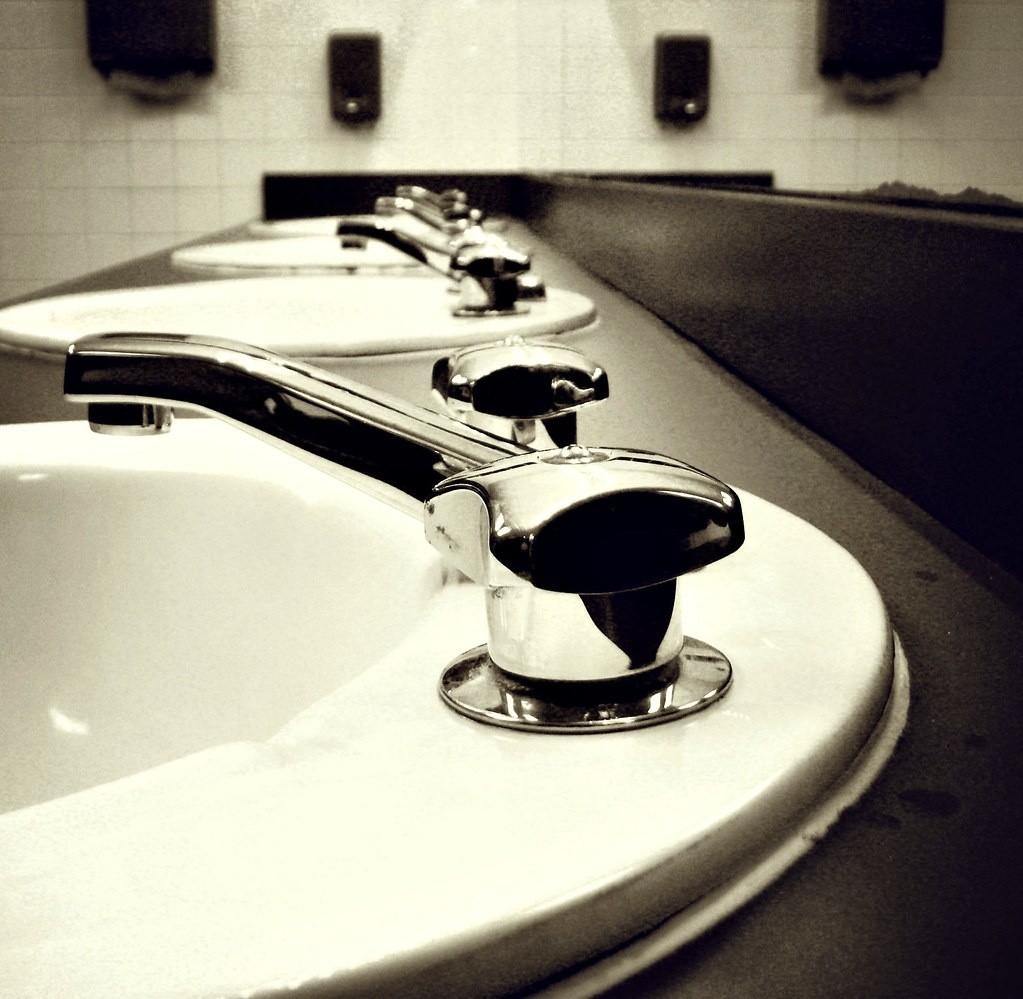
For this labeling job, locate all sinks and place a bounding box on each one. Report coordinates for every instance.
[0,416,910,999]
[0,276,600,357]
[170,236,522,273]
[245,214,511,235]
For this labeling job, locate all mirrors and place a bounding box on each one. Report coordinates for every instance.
[521,0,1023,210]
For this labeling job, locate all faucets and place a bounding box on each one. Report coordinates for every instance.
[394,185,468,211]
[374,196,470,234]
[61,331,746,734]
[332,217,547,317]
